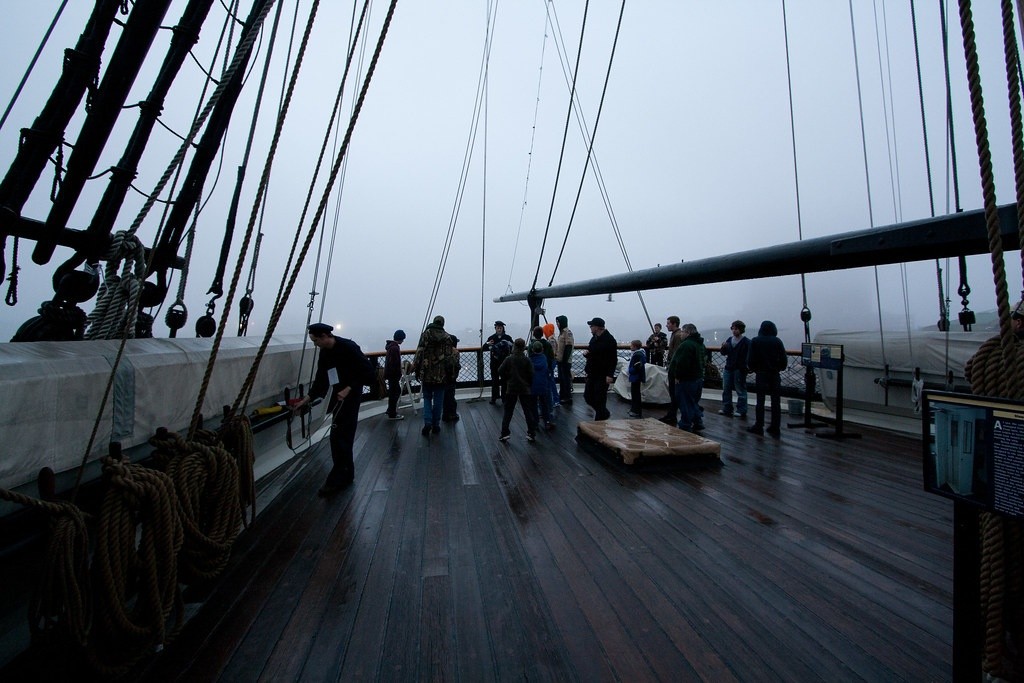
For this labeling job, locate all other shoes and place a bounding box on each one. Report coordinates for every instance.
[692,424,705,430]
[489,397,497,404]
[734,412,743,416]
[560,398,571,403]
[387,414,404,420]
[552,401,561,408]
[318,478,345,496]
[718,409,731,414]
[432,425,440,433]
[443,414,459,421]
[422,424,432,434]
[658,415,676,422]
[545,422,556,429]
[629,411,642,417]
[766,427,779,434]
[747,427,763,435]
[526,434,535,441]
[499,433,511,441]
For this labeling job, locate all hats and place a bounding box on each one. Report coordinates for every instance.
[513,339,525,353]
[588,318,605,327]
[394,330,405,340]
[308,323,333,334]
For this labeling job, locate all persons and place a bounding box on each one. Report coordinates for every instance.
[527,326,554,418]
[498,339,537,441]
[555,315,574,403]
[543,323,560,407]
[627,339,646,416]
[668,323,708,430]
[583,317,618,421]
[414,315,453,434]
[384,330,406,420]
[529,341,552,428]
[442,335,461,419]
[748,322,788,433]
[659,316,682,423]
[482,321,514,404]
[718,320,751,417]
[646,323,668,367]
[292,323,374,493]
